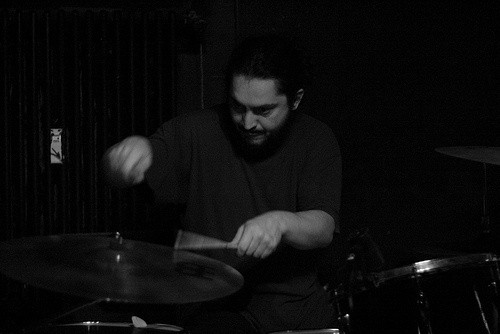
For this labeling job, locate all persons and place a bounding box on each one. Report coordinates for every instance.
[106,41,337,334]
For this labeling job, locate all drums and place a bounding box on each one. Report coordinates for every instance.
[347,254,500,334]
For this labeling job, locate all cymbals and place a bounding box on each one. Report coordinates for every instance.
[0,235,245,308]
